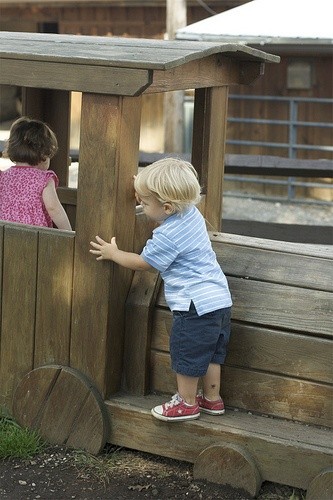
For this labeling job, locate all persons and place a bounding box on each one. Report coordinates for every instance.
[0,116,72,231]
[88,156,234,422]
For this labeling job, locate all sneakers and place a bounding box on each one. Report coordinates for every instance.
[196,395,225,415]
[151,392,201,422]
